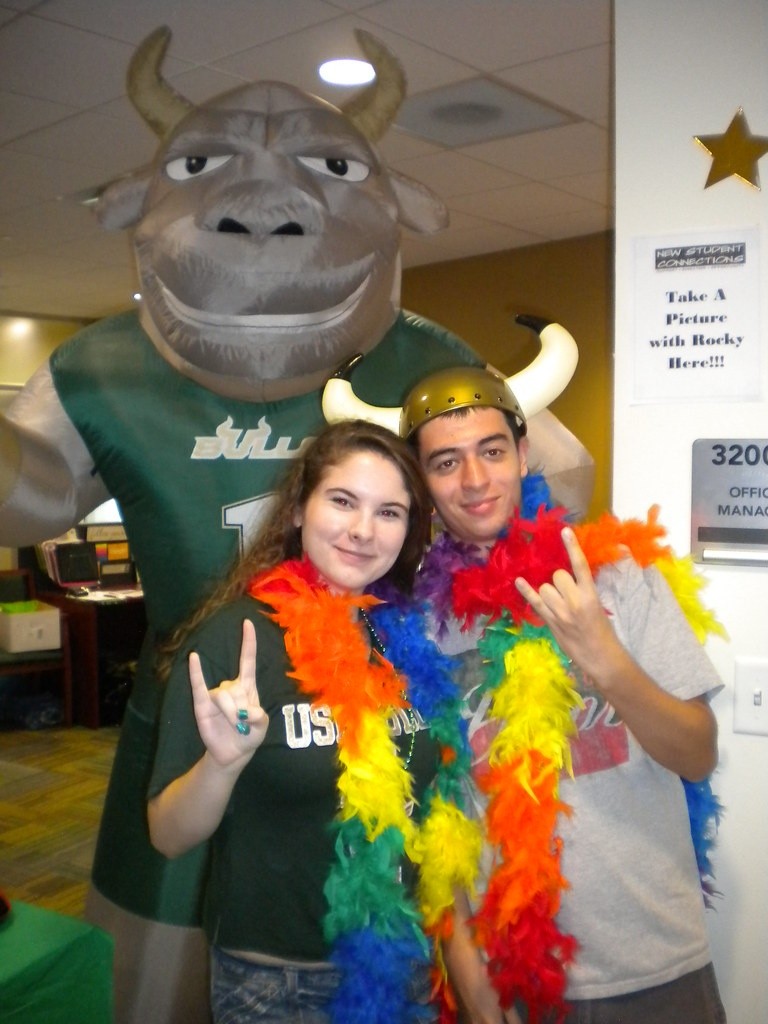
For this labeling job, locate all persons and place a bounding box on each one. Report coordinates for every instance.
[405,366,728,1024]
[147,420,444,1024]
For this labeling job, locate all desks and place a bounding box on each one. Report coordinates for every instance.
[40,586,146,728]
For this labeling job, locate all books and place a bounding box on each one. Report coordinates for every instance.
[36,528,99,588]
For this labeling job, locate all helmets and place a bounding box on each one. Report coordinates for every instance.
[321,314,579,441]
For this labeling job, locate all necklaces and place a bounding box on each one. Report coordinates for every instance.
[252,474,727,1024]
[351,607,416,770]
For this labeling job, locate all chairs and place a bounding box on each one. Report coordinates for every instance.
[0,567,73,729]
